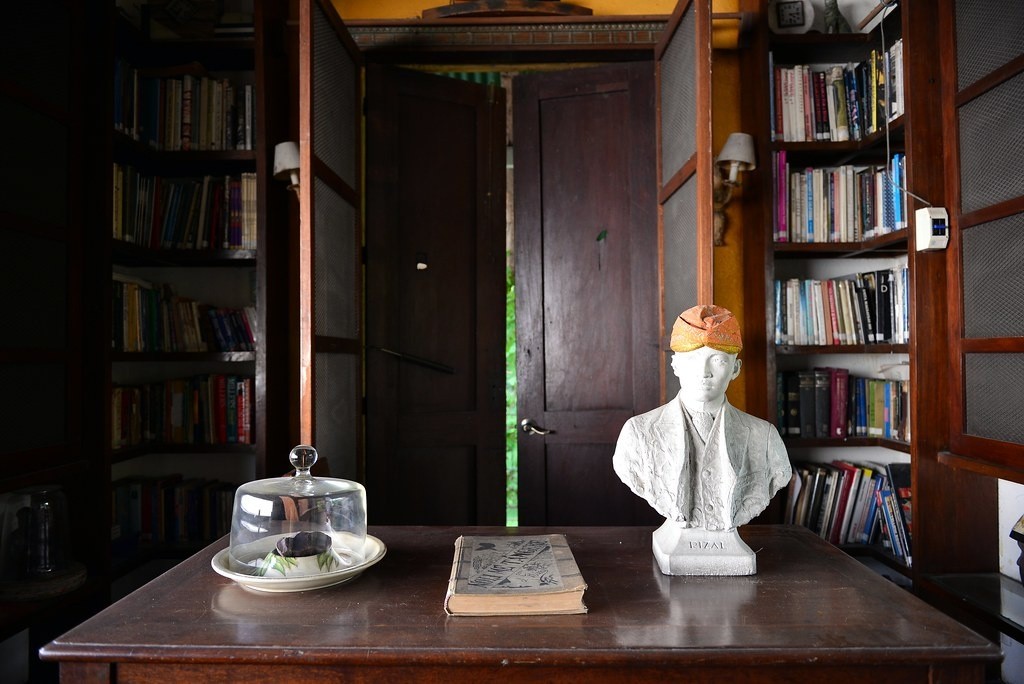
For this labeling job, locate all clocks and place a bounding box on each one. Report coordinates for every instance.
[776,1,805,28]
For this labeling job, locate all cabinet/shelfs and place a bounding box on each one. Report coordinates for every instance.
[734,13,1024,684]
[0,575,103,684]
[96,1,291,609]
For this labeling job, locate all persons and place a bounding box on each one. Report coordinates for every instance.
[612,305,792,532]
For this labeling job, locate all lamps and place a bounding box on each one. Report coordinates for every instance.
[712,133,757,206]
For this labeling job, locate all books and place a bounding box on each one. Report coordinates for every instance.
[110,55,257,584]
[768,37,912,567]
[444,533,588,616]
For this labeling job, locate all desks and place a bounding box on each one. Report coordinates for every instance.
[37,527,1004,684]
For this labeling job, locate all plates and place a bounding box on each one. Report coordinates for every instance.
[212,531,387,592]
